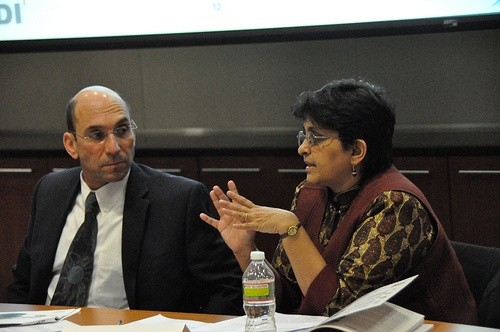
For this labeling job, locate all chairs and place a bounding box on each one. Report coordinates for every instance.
[451,242,500,329]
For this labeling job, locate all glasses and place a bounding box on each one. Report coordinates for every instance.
[67,119,138,143]
[297,130,342,145]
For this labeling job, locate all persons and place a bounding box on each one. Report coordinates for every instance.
[199,79,480,326]
[5,85,246,316]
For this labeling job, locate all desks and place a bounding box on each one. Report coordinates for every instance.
[0,303,500,332]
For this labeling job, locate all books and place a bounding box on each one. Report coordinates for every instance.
[196,275,434,332]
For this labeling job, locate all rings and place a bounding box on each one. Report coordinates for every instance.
[244,213,248,222]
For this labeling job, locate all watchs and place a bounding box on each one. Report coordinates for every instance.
[279,222,302,238]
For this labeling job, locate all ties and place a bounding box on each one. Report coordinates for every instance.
[50,191,100,306]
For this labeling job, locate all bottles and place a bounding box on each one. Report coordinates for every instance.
[242,251,277,332]
